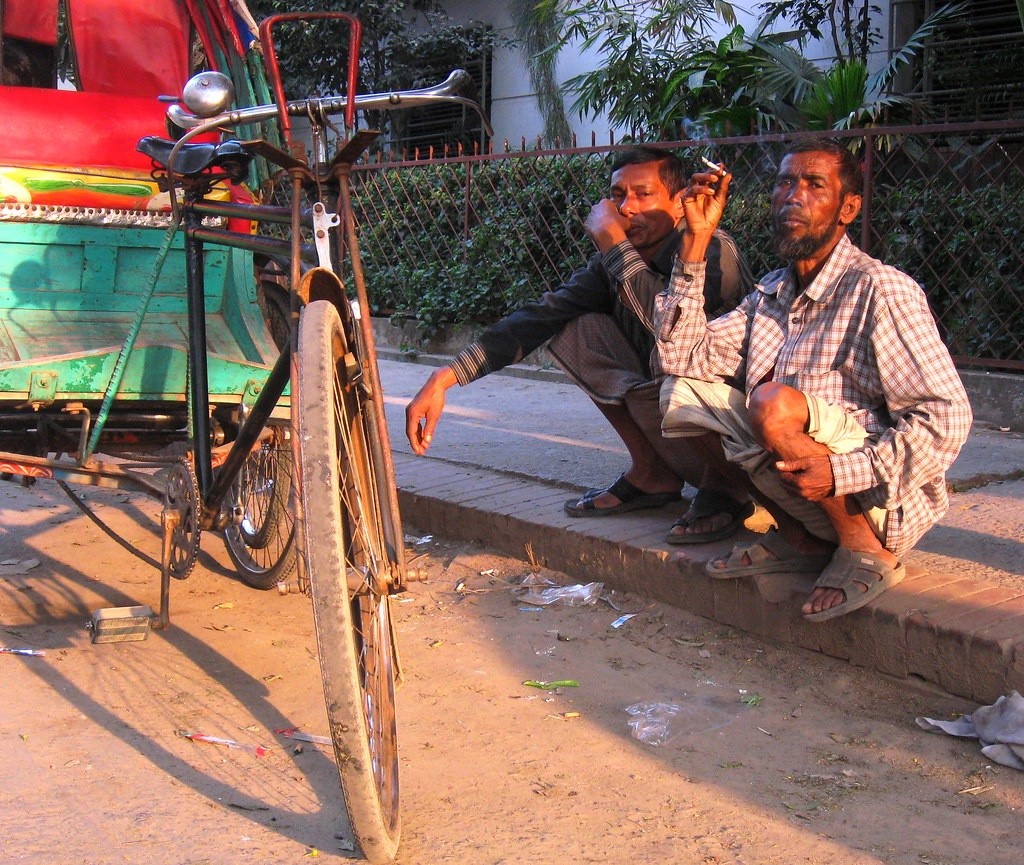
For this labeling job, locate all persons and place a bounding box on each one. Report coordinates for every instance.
[406,147,754,544]
[649,137,973,624]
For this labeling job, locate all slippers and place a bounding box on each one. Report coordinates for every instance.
[667,487,757,543]
[564,471,682,516]
[705,524,832,579]
[801,547,906,622]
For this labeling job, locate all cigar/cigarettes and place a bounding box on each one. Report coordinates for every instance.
[702,157,727,176]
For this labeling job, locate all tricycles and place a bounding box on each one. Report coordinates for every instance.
[1,0,499,865]
